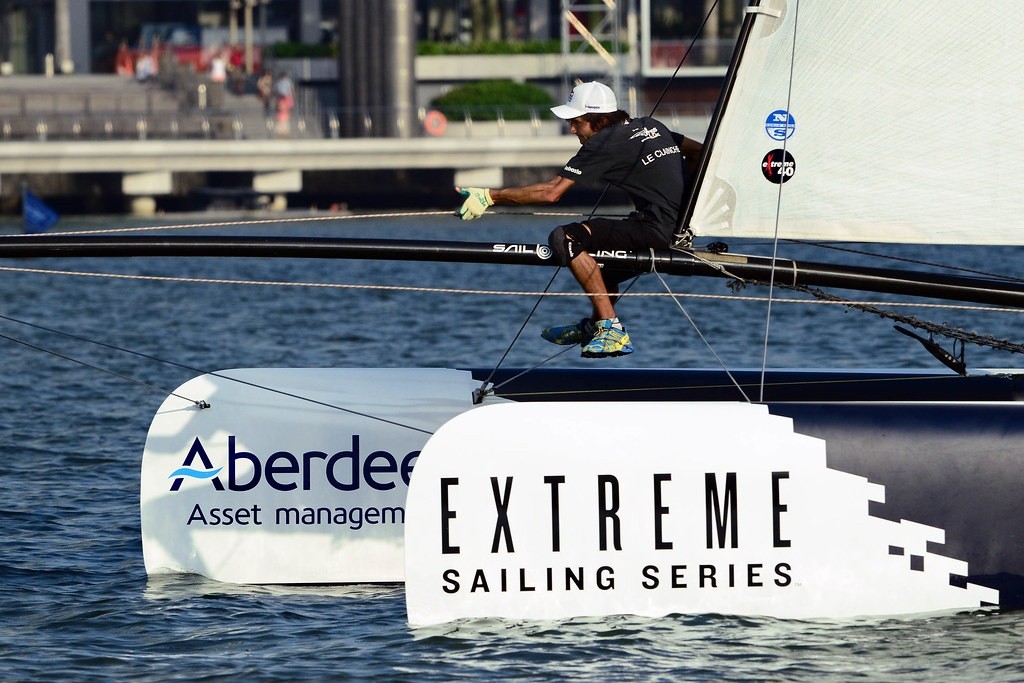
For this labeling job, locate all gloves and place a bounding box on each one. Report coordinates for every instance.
[453,186,495,220]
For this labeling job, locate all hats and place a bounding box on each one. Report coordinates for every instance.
[550,80,617,119]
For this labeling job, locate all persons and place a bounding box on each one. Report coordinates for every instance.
[257,71,294,124]
[134,49,155,84]
[452,81,703,358]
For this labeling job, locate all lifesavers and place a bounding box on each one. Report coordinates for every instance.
[422,110,448,137]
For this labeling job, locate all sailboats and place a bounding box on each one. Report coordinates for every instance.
[0,1,1024,621]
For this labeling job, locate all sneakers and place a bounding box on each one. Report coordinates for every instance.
[581,319,634,358]
[541,318,598,345]
